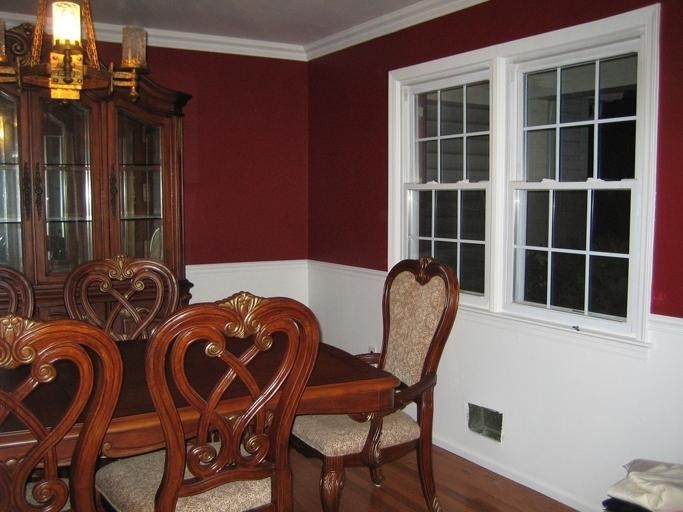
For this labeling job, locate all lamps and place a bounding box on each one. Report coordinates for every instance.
[1,1,149,102]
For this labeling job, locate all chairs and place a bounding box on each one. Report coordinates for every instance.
[61,256,179,339]
[0,265,32,317]
[290,256,460,510]
[93,291,323,510]
[0,310,122,509]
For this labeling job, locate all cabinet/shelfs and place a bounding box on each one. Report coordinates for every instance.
[0,22,193,340]
